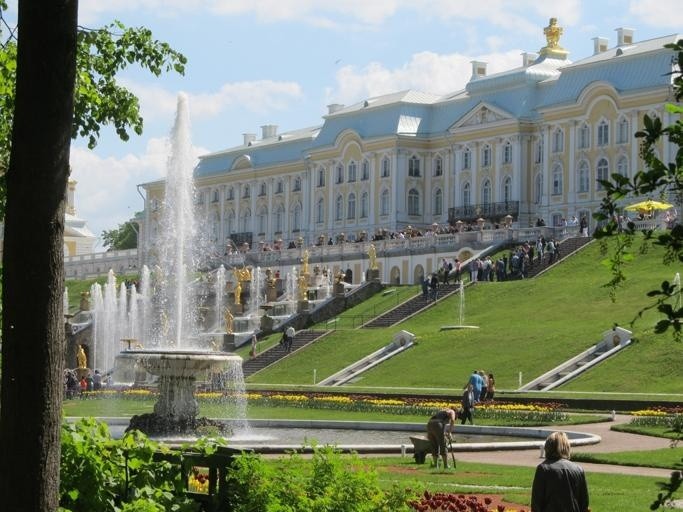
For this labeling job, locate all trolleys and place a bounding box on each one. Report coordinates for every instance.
[410,434,457,463]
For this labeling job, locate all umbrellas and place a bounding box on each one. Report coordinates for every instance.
[623,197,675,213]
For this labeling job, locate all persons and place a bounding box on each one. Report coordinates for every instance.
[530,431,589,512]
[461,384,475,425]
[262,222,473,252]
[570,215,579,237]
[578,217,588,237]
[463,370,485,403]
[248,335,257,360]
[485,373,495,400]
[63,369,112,401]
[285,324,295,353]
[557,218,567,235]
[279,328,288,352]
[477,370,488,401]
[419,233,560,304]
[427,406,458,468]
[535,218,545,227]
